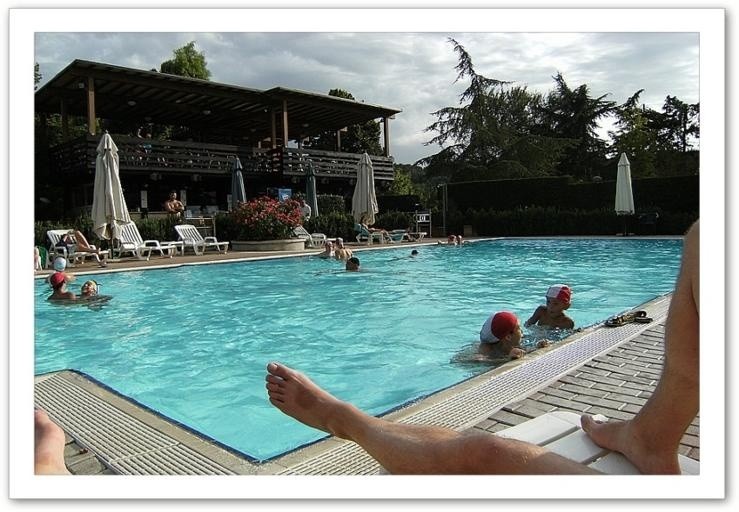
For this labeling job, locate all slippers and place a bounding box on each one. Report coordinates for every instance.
[605,315,635,327]
[627,311,653,323]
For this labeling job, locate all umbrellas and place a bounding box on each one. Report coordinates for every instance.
[90,128,131,259]
[351,152,379,229]
[305,162,319,221]
[609,150,639,240]
[231,157,248,211]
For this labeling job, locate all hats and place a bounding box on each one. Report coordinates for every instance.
[545,284,571,305]
[50,273,64,286]
[54,257,66,272]
[480,311,517,342]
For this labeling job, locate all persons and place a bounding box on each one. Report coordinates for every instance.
[34,229,113,311]
[319,238,360,270]
[520,281,578,336]
[451,311,550,366]
[163,190,185,214]
[129,127,148,168]
[264,217,700,474]
[299,199,312,222]
[34,406,76,474]
[447,235,457,246]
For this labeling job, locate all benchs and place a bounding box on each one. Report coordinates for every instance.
[117,221,176,262]
[292,224,337,248]
[159,224,229,255]
[358,226,427,244]
[47,229,107,267]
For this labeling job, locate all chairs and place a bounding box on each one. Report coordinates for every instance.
[491,409,699,475]
